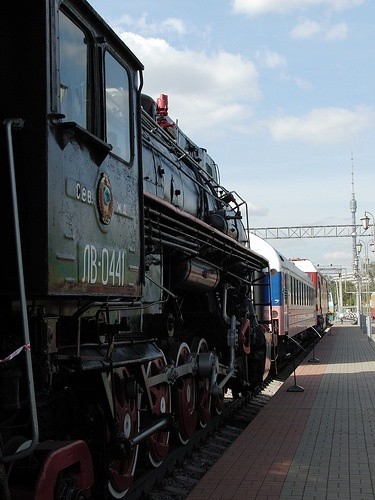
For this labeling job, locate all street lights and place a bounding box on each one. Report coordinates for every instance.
[353,211,375,341]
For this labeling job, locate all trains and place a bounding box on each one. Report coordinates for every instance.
[0,0,334,500]
